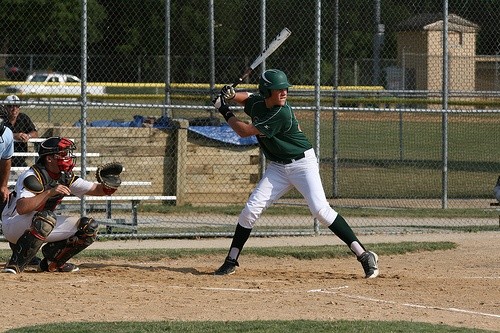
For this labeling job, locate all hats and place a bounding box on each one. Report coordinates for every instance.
[4,95,20,101]
[35,137,71,163]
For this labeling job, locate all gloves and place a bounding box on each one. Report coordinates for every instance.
[220,84,236,101]
[213,95,229,112]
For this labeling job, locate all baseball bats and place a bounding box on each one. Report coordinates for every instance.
[231,27,293,91]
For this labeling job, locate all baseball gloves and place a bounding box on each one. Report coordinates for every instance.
[96,162,123,191]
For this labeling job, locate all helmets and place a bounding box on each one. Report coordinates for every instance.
[259,69,292,97]
[0,104,10,136]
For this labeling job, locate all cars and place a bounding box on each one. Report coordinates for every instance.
[6,73,107,97]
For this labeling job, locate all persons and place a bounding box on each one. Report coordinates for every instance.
[2,137,122,274]
[2,95,37,167]
[0,104,14,224]
[213,69,379,279]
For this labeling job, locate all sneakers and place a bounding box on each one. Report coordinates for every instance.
[40,257,79,272]
[5,263,21,274]
[214,256,239,275]
[358,250,379,279]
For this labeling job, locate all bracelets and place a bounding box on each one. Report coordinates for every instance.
[28,134,32,137]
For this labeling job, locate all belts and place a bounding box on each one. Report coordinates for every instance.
[272,152,305,165]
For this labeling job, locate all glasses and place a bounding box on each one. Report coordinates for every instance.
[7,105,20,108]
